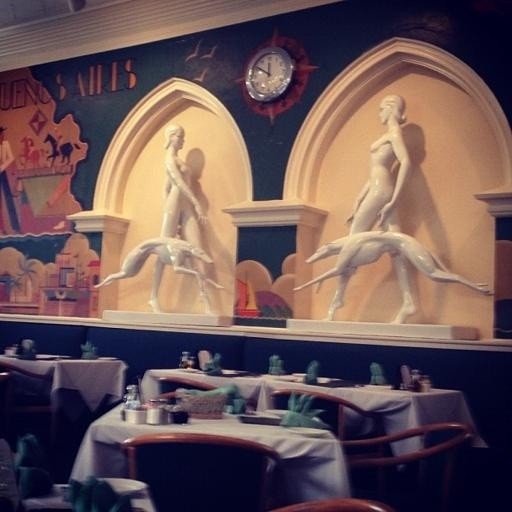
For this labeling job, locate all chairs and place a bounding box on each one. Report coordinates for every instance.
[340,422,473,511]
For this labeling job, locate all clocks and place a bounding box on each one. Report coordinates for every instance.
[245,46,297,103]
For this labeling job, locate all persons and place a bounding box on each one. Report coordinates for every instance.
[0,126,22,236]
[148,122,217,313]
[322,95,417,325]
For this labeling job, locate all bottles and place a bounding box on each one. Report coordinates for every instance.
[125,384,172,426]
[180,351,196,370]
